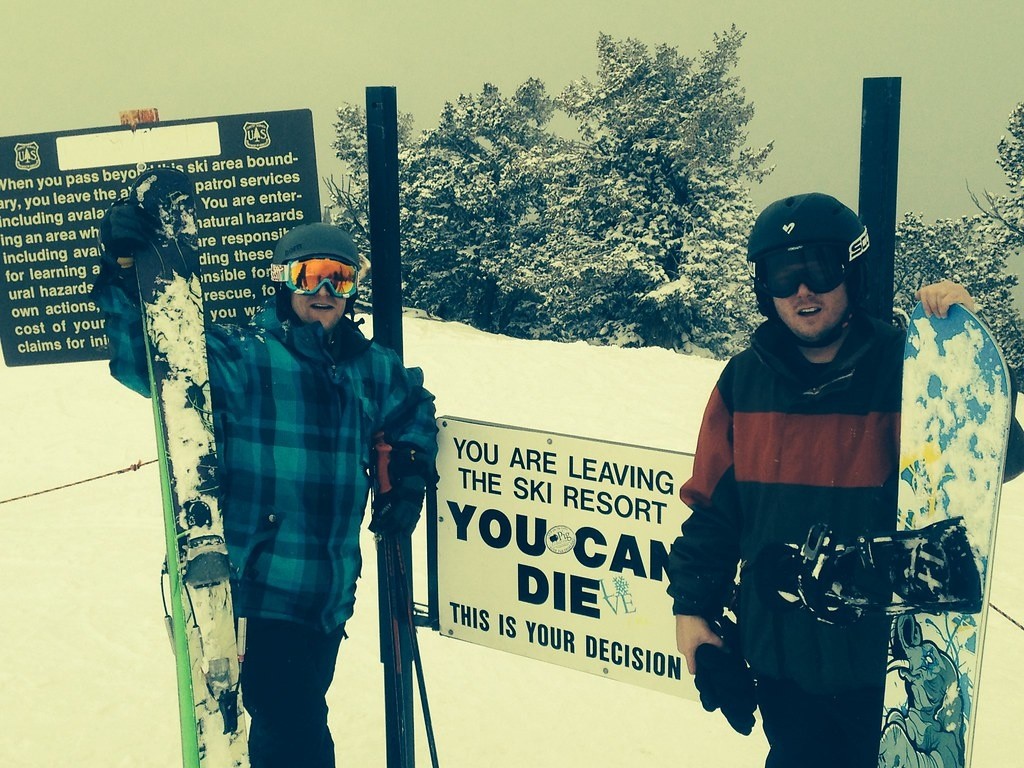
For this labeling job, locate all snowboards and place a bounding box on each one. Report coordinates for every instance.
[874,294,1015,768]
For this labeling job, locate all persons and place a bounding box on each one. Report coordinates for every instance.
[659,191,1024,768]
[91,196,440,768]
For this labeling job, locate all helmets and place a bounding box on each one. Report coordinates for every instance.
[747,193,873,303]
[272,222,362,271]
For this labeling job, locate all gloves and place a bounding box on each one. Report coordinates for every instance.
[101,199,163,259]
[694,615,756,736]
[368,449,432,538]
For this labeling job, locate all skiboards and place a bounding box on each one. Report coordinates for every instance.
[123,166,255,768]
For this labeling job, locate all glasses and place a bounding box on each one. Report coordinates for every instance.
[284,253,358,298]
[755,241,853,299]
